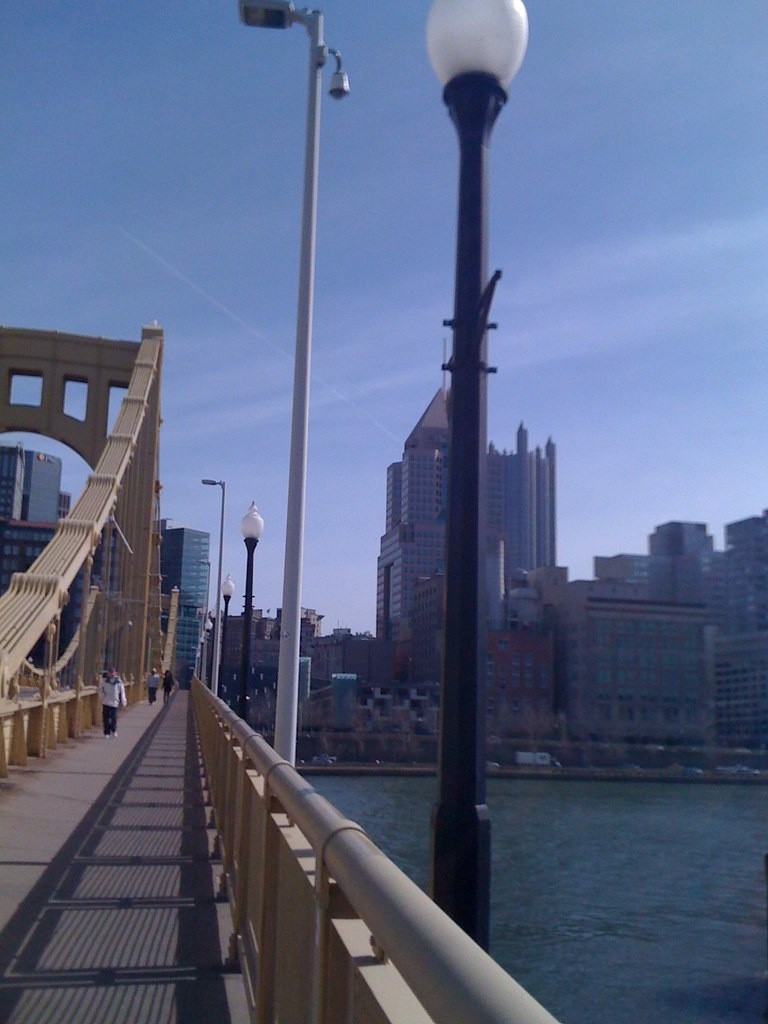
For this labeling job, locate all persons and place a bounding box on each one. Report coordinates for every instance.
[145,668,160,705]
[163,669,174,703]
[99,667,128,738]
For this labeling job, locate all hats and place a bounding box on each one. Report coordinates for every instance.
[109,668,115,672]
[152,668,157,671]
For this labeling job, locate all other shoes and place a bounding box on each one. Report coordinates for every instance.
[113,732,117,738]
[150,703,154,705]
[105,735,110,739]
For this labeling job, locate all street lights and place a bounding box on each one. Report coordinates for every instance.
[236,0,352,776]
[193,558,238,695]
[238,499,265,722]
[199,477,227,693]
[427,1,532,971]
